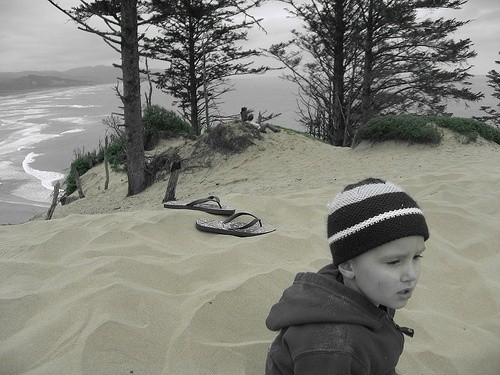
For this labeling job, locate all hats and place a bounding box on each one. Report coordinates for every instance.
[327,177,429,266]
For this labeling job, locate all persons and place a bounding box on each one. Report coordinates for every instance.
[263,178,431,374]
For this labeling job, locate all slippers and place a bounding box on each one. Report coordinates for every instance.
[195,212,276,237]
[165,193,236,215]
